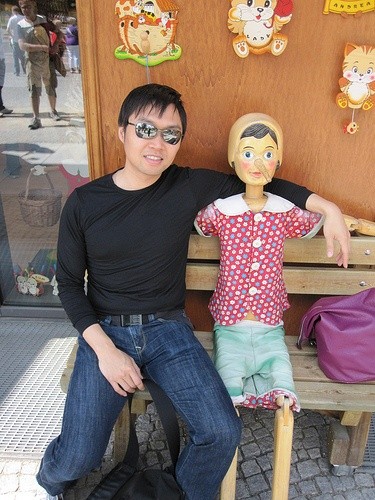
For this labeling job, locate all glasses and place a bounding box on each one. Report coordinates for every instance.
[126,121,183,145]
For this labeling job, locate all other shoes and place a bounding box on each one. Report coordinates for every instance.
[0,109,13,114]
[48,110,60,120]
[28,118,43,128]
[0,113,3,117]
[2,169,20,179]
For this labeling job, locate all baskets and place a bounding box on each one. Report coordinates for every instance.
[16,167,62,227]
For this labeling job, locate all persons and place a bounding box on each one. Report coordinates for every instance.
[35,84,350,500]
[0,0,59,130]
[2,121,48,178]
[193,112,375,500]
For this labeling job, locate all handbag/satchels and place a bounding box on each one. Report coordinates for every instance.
[87,466,185,500]
[295,288,375,383]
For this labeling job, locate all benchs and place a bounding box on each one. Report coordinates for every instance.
[112,229,375,474]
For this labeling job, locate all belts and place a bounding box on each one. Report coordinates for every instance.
[101,309,193,330]
